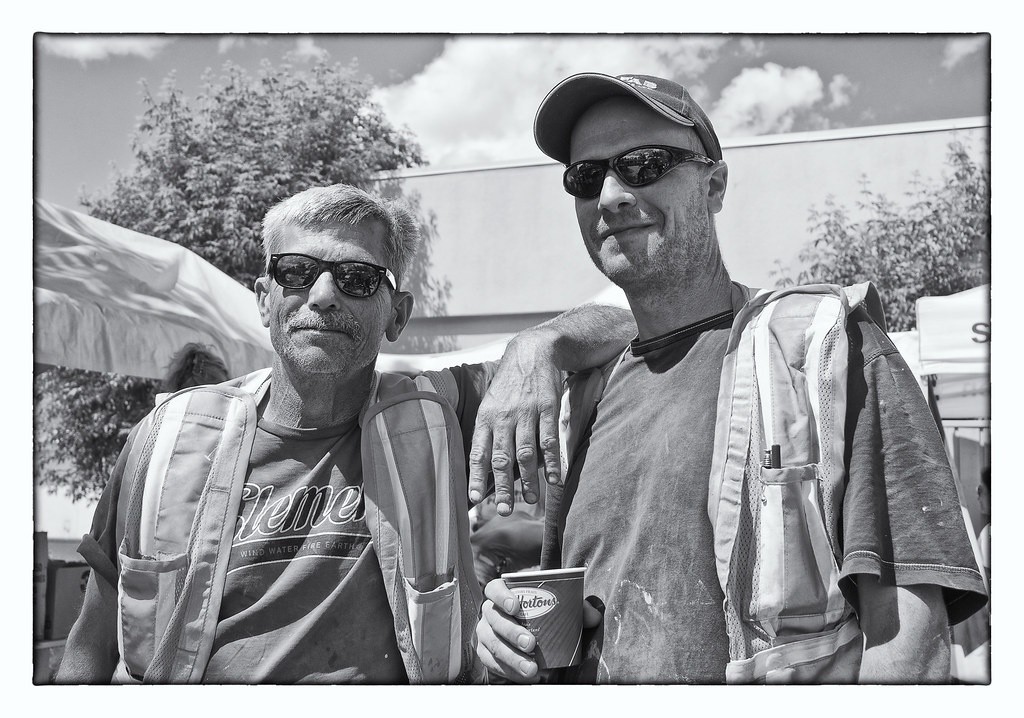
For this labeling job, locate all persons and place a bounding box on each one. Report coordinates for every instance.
[464,458,991,685]
[471,73,987,684]
[52,181,639,684]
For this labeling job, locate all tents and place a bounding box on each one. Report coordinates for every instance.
[35,199,989,456]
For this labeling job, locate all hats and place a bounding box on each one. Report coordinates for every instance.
[533,73,722,164]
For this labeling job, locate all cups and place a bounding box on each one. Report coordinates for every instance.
[502,569,583,668]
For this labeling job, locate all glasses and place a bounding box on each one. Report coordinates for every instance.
[267,253,396,298]
[563,145,715,199]
[978,485,986,495]
